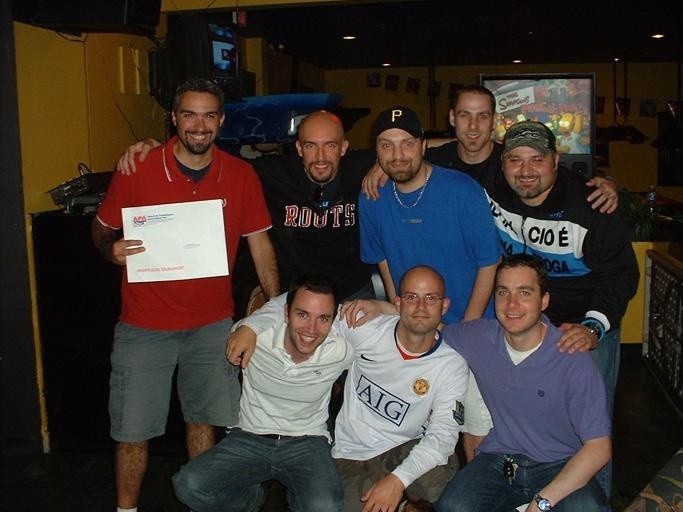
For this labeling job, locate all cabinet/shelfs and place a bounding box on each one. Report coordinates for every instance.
[619,184,682,344]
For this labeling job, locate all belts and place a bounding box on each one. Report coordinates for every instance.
[258,433,284,441]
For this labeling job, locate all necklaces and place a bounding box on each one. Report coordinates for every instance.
[392,165,429,208]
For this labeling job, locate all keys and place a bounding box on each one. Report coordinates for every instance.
[504,463,515,485]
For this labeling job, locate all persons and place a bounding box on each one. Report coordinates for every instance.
[478,120,639,501]
[339,254,613,512]
[170,277,354,512]
[92,81,278,512]
[360,88,619,214]
[116,111,380,442]
[225,267,468,512]
[356,108,500,462]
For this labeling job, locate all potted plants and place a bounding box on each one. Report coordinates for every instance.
[613,194,682,240]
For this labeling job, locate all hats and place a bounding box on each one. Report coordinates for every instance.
[367,105,423,144]
[500,118,558,156]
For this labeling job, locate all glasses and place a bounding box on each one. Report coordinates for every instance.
[503,155,547,163]
[398,291,447,304]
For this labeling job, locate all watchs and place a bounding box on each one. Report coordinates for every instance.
[532,494,554,512]
[585,324,601,339]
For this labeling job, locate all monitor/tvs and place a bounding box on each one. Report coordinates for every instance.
[149,8,243,109]
[10,0,161,38]
[481,74,594,162]
